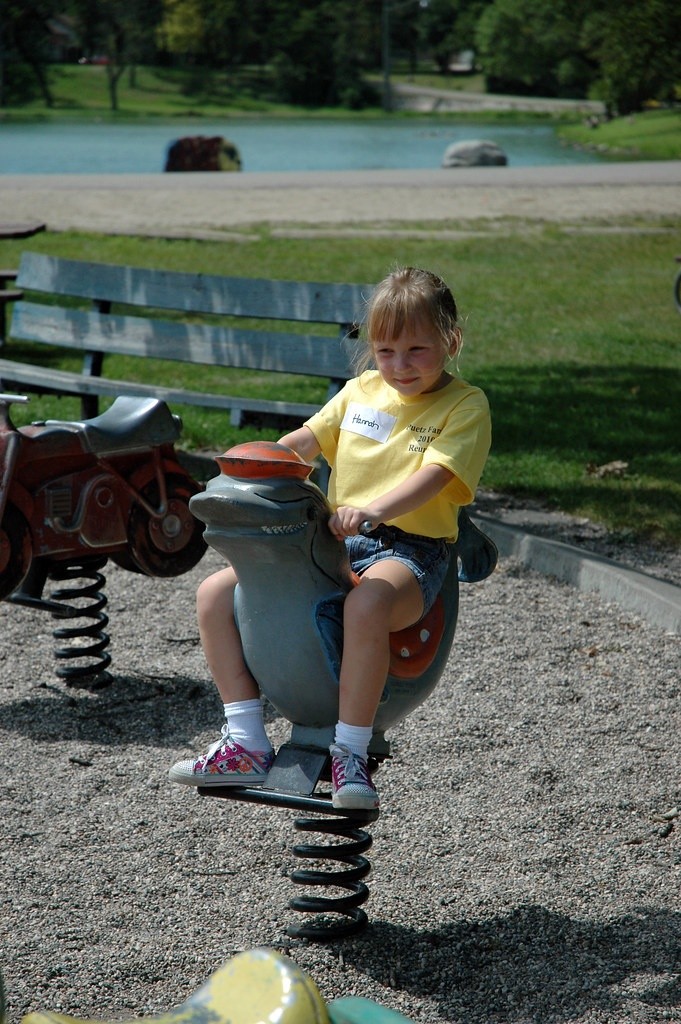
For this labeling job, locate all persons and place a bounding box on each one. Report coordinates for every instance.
[171,266,489,811]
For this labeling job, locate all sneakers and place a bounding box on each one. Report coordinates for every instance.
[325,741,381,810]
[168,724,277,787]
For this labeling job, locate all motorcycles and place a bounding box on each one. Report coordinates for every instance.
[1,389,207,602]
